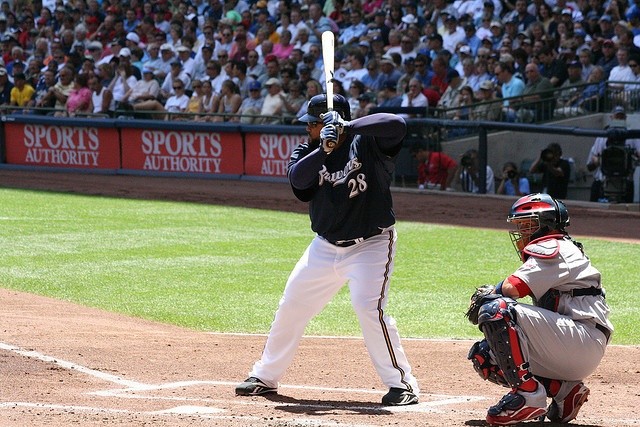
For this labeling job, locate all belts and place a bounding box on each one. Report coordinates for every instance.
[326,230,382,247]
[596,323,611,341]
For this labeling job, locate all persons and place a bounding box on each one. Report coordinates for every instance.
[410,142,457,191]
[529,143,570,199]
[235,94,421,406]
[586,106,640,202]
[468,193,614,425]
[451,150,495,194]
[307,1,493,138]
[493,0,640,130]
[0,0,307,125]
[496,162,530,196]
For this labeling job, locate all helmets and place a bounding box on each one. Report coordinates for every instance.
[506,193,570,261]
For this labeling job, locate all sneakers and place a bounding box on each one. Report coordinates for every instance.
[235,377,277,396]
[486,383,548,427]
[382,387,419,406]
[547,381,590,423]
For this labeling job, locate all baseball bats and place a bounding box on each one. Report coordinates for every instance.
[322,30,338,148]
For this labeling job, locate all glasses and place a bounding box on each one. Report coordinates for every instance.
[350,86,357,89]
[89,50,95,53]
[478,55,485,58]
[223,34,230,37]
[75,48,83,51]
[54,56,63,59]
[526,70,533,74]
[604,45,613,48]
[193,86,200,88]
[495,71,502,76]
[173,87,182,90]
[529,55,538,58]
[415,65,423,67]
[310,50,317,53]
[307,122,323,128]
[206,66,211,69]
[629,65,637,67]
[250,89,258,91]
[218,55,226,58]
[204,32,211,35]
[401,41,410,44]
[502,42,511,45]
[266,86,273,88]
[367,68,374,71]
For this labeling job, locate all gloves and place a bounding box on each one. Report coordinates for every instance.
[319,124,339,155]
[320,111,344,134]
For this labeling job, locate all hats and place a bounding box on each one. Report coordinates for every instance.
[443,70,459,83]
[404,57,414,65]
[234,34,246,40]
[587,10,599,20]
[611,106,625,114]
[518,30,529,37]
[613,19,628,29]
[482,36,493,43]
[358,40,370,47]
[202,44,212,50]
[561,8,571,15]
[119,47,131,57]
[603,39,614,45]
[401,14,418,24]
[445,14,456,22]
[480,80,491,89]
[184,13,198,23]
[12,73,24,79]
[567,60,582,68]
[598,14,611,23]
[142,66,154,73]
[13,59,21,66]
[427,33,442,40]
[89,41,102,49]
[160,43,171,50]
[460,46,472,54]
[552,6,561,13]
[74,41,84,47]
[380,80,397,90]
[298,93,351,122]
[249,81,261,89]
[177,46,189,52]
[126,32,139,43]
[489,20,502,28]
[170,57,183,66]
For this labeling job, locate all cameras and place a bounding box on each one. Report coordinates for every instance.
[507,170,514,178]
[540,148,555,163]
[462,94,469,101]
[461,154,474,169]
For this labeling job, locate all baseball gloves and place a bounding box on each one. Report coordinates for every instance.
[465,285,492,324]
